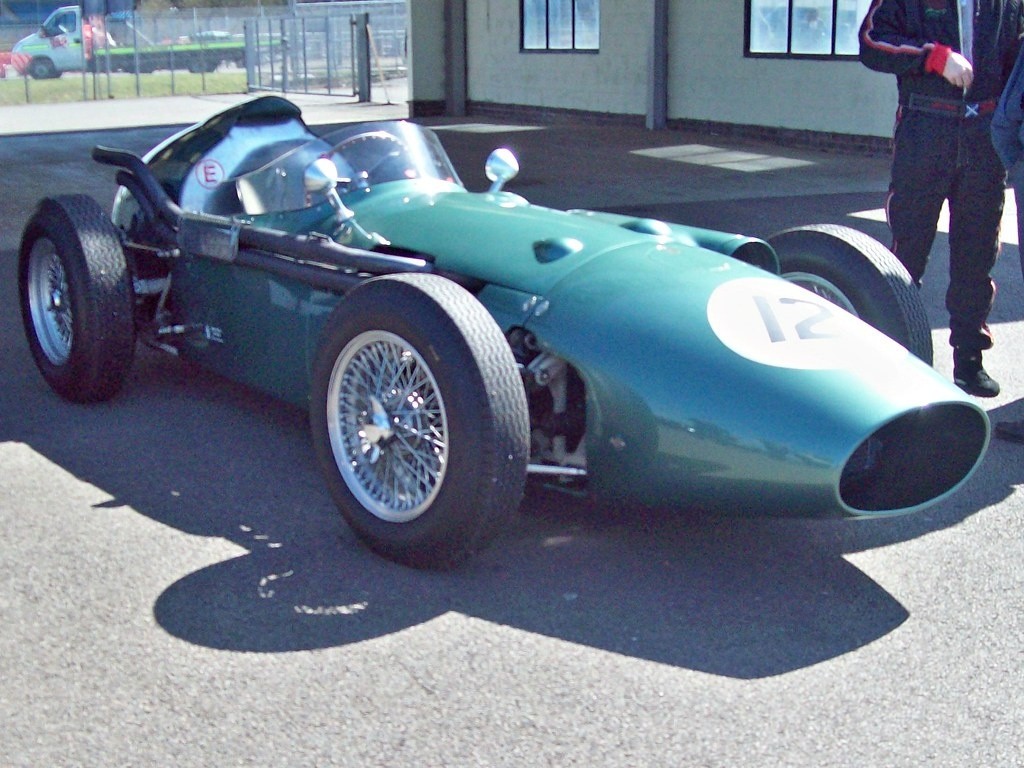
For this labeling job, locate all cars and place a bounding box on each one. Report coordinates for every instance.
[16,95,991,572]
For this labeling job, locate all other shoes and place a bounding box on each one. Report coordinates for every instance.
[951,345,1000,399]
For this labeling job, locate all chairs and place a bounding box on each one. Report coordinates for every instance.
[220,139,359,216]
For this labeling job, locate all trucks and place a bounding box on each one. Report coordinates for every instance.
[9,5,290,81]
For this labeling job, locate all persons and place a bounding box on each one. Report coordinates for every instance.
[859,0,1024,397]
[991,41,1024,281]
